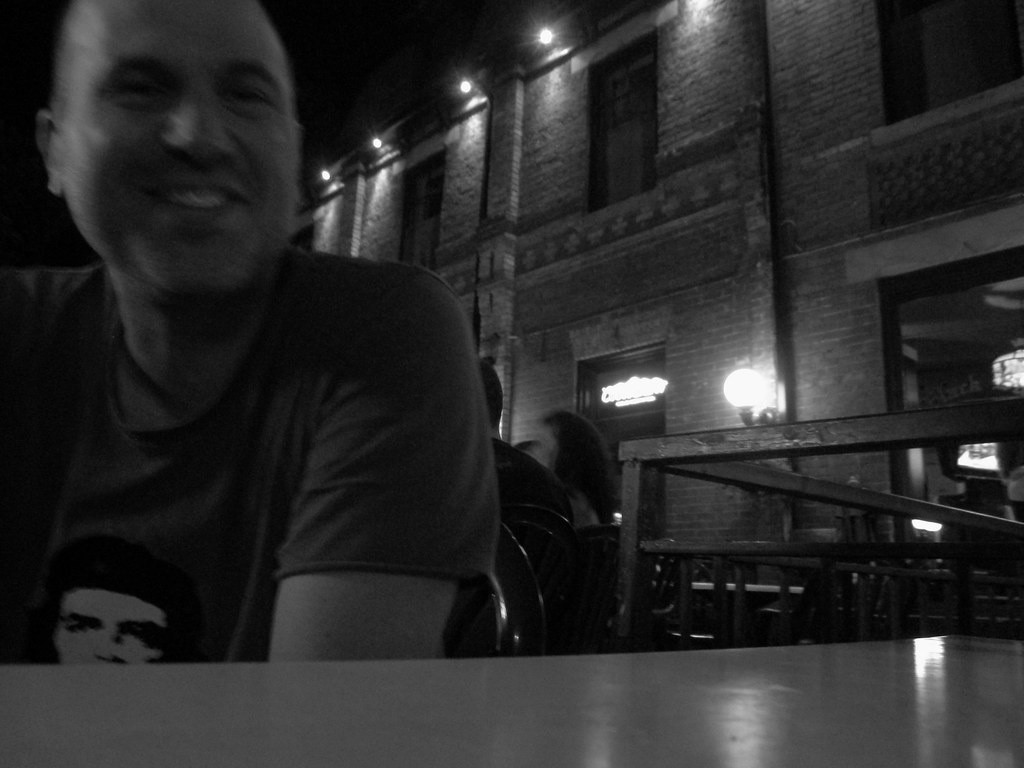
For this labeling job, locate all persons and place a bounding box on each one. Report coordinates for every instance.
[0,1,502,667]
[478,360,615,528]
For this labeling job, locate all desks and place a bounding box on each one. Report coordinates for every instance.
[0,636,1024,768]
